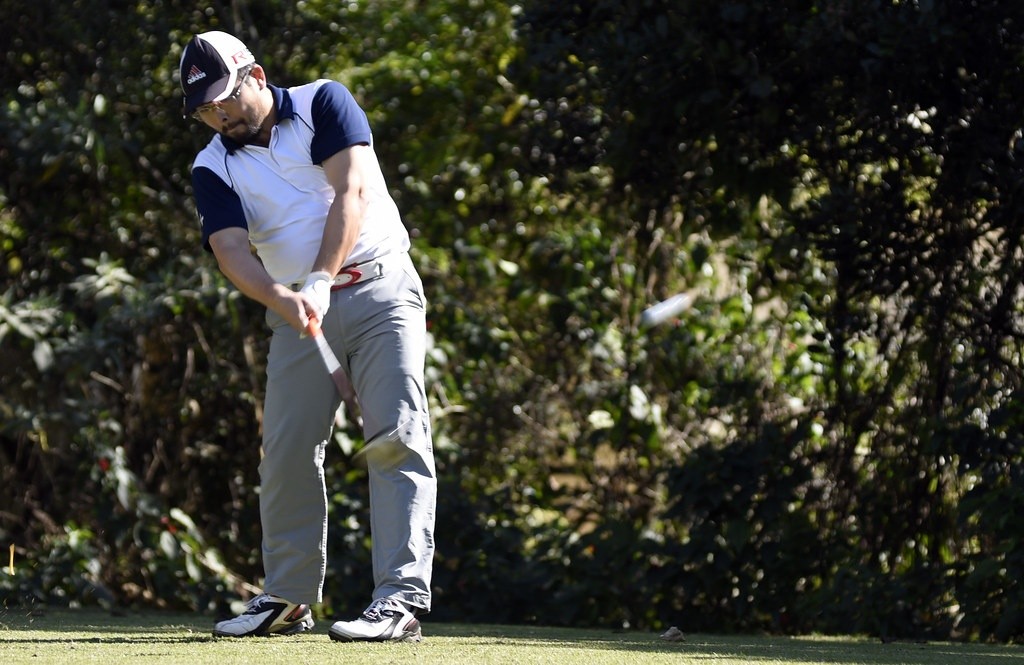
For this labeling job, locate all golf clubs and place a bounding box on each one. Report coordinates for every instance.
[303,313,413,470]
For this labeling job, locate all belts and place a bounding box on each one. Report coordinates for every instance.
[283,252,396,293]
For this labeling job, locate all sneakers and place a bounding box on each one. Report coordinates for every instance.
[328,598,422,643]
[213,594,315,638]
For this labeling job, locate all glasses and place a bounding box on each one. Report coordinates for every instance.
[192,66,250,122]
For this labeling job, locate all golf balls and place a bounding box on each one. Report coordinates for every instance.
[648,289,693,325]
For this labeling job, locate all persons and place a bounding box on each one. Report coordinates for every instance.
[179,30,438,643]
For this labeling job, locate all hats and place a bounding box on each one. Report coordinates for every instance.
[180,31,255,118]
